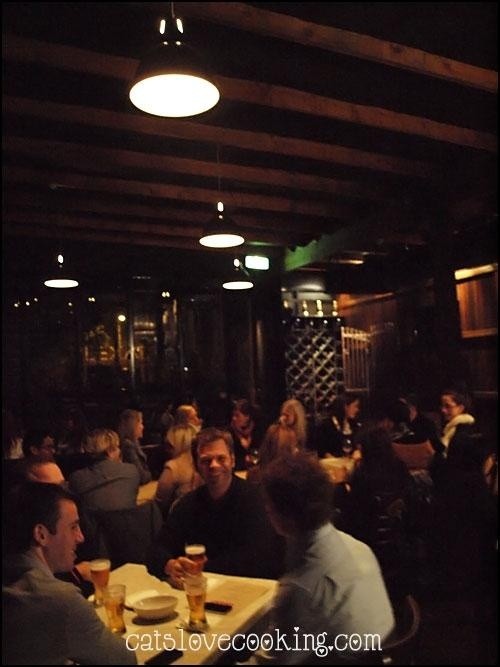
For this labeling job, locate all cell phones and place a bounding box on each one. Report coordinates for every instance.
[143,647,183,666]
[204,601,232,614]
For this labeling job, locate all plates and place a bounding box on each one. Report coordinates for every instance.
[125,589,158,610]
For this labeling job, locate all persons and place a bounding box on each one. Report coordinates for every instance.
[0,479,138,667]
[158,389,200,430]
[395,390,441,451]
[267,399,307,449]
[113,410,154,486]
[233,452,396,666]
[65,429,141,569]
[345,397,412,521]
[312,390,369,497]
[18,458,66,488]
[381,400,436,468]
[244,421,315,579]
[1,408,22,483]
[176,406,200,431]
[50,397,90,449]
[126,337,157,366]
[403,378,487,553]
[224,399,270,471]
[22,427,57,463]
[153,421,204,524]
[145,427,284,590]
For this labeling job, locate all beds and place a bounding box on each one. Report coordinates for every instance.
[67,562,282,667]
[235,457,352,485]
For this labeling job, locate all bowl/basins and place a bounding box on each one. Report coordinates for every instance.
[132,595,178,622]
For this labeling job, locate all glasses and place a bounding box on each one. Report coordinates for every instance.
[438,403,457,408]
[37,443,55,450]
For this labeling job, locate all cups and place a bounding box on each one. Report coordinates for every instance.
[250,449,260,468]
[185,545,206,576]
[90,558,111,606]
[104,584,127,637]
[184,578,212,634]
[343,439,352,462]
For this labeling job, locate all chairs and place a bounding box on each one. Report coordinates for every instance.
[363,593,423,667]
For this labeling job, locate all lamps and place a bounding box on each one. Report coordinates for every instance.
[44,262,78,289]
[198,129,246,249]
[244,248,271,270]
[222,267,254,290]
[127,0,221,117]
